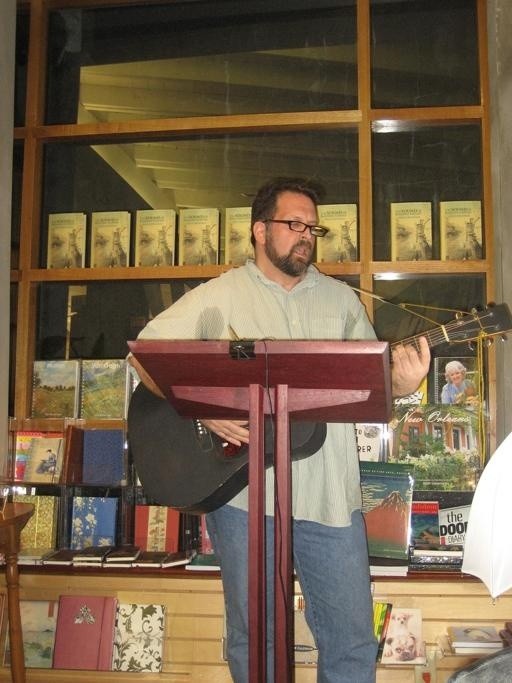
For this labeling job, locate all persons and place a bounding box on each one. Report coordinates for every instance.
[124,172,432,683]
[124,177,432,683]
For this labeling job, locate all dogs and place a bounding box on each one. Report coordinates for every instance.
[392,612,414,634]
[384,634,416,662]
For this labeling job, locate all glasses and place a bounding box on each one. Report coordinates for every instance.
[260,212,330,240]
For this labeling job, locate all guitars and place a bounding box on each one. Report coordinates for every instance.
[128,303,511,516]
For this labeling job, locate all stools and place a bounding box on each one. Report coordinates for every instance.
[1,499,39,683]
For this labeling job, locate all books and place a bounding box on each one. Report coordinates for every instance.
[7,358,220,571]
[4,595,165,673]
[47,203,482,269]
[294,596,508,667]
[355,355,487,579]
